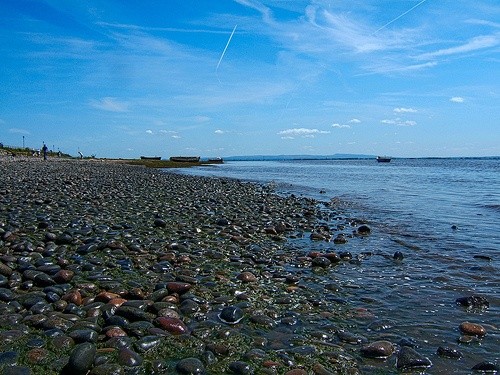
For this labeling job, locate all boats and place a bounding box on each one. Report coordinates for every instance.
[140,156,161,160]
[377,158,391,162]
[170,156,201,162]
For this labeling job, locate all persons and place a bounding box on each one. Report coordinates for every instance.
[42,144,48,160]
[57,151,62,158]
[78,151,84,160]
[11,151,16,158]
[31,147,35,157]
[50,150,53,156]
[37,148,41,157]
